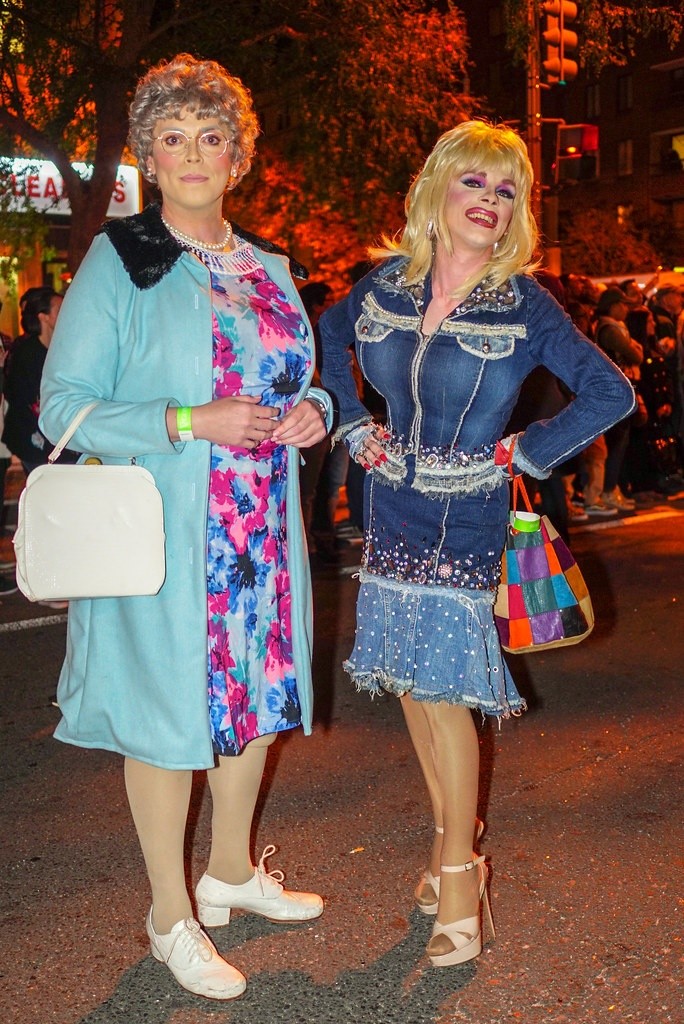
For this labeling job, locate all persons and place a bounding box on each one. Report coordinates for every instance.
[319,121,637,967]
[38,54,334,1000]
[0,258,683,573]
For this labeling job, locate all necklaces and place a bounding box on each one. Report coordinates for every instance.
[156,217,233,249]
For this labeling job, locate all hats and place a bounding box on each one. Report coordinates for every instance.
[600,287,638,306]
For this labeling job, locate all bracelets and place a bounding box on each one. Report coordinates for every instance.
[176,406,196,443]
[308,397,328,419]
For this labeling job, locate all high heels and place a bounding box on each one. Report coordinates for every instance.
[425,850,497,966]
[412,817,483,915]
[194,848,324,927]
[145,904,247,1000]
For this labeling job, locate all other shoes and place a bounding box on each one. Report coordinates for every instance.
[307,526,350,549]
[570,490,585,506]
[580,499,618,515]
[601,486,636,511]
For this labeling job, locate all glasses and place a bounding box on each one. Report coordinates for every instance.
[150,128,235,159]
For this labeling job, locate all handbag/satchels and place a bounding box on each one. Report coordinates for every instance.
[11,400,166,602]
[496,434,595,653]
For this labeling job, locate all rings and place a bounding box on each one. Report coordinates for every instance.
[372,426,380,436]
[363,448,370,455]
[356,455,360,460]
